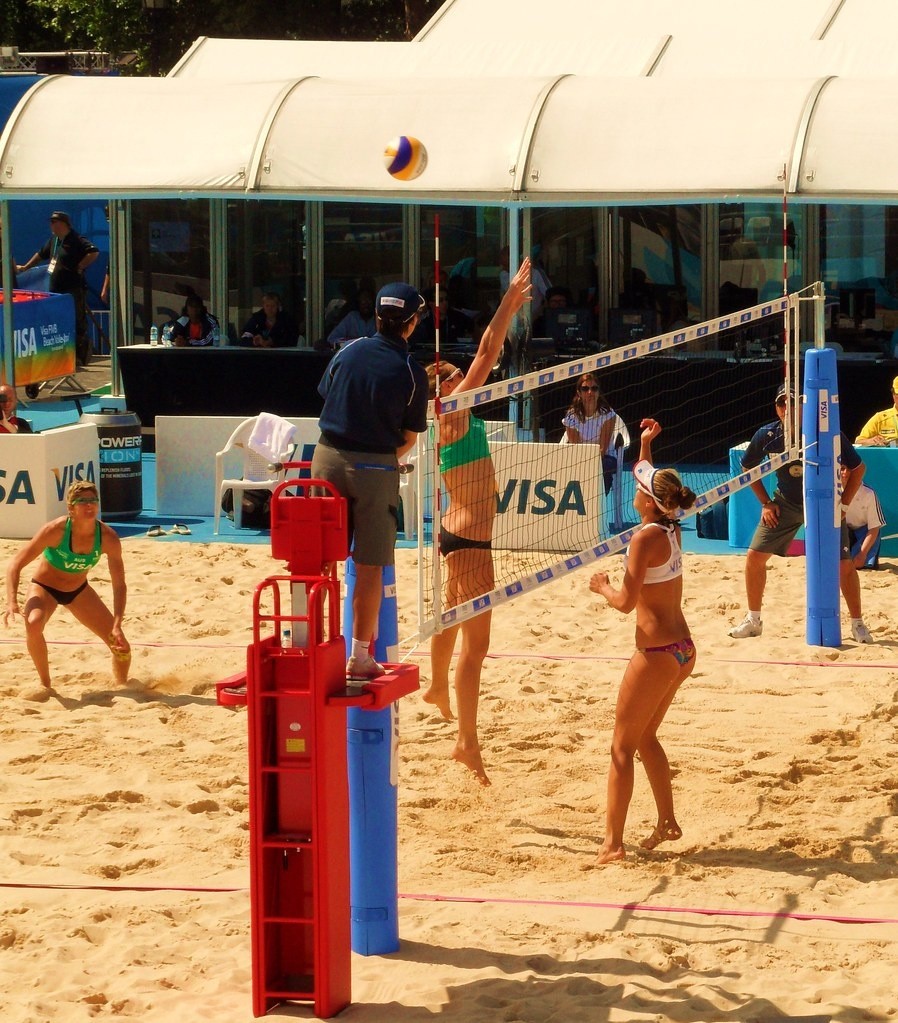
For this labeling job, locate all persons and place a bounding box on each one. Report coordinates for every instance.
[309,282,430,681]
[425,257,532,786]
[0,478,131,689]
[654,295,707,351]
[327,288,376,347]
[100,263,110,302]
[449,240,499,284]
[237,291,300,348]
[841,462,887,571]
[531,286,567,339]
[420,286,474,344]
[15,211,99,366]
[727,382,874,643]
[854,376,898,447]
[586,418,697,867]
[0,383,34,434]
[159,294,220,347]
[561,372,617,497]
[500,247,553,321]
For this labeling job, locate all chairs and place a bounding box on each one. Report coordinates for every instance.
[558,414,631,529]
[213,415,293,537]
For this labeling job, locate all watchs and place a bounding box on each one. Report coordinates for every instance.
[763,500,773,507]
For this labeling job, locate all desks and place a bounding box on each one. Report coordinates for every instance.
[729,440,898,556]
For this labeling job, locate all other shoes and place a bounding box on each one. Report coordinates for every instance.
[26,383,39,399]
[79,339,93,366]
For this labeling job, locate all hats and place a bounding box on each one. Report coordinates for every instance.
[774,382,803,401]
[48,212,72,226]
[892,376,898,394]
[632,459,676,514]
[375,282,426,324]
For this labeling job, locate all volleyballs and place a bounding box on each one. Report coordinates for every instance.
[383,135,429,181]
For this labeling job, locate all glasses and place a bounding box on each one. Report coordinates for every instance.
[430,296,450,302]
[69,496,99,505]
[580,385,600,392]
[550,300,568,305]
[774,398,795,408]
[635,481,662,502]
[435,367,464,393]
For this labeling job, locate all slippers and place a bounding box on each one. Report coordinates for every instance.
[170,524,191,535]
[146,525,166,536]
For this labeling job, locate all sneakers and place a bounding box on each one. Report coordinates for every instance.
[727,618,763,637]
[345,654,385,681]
[851,621,872,643]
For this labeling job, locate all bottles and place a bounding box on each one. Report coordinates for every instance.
[163,323,170,346]
[150,323,158,346]
